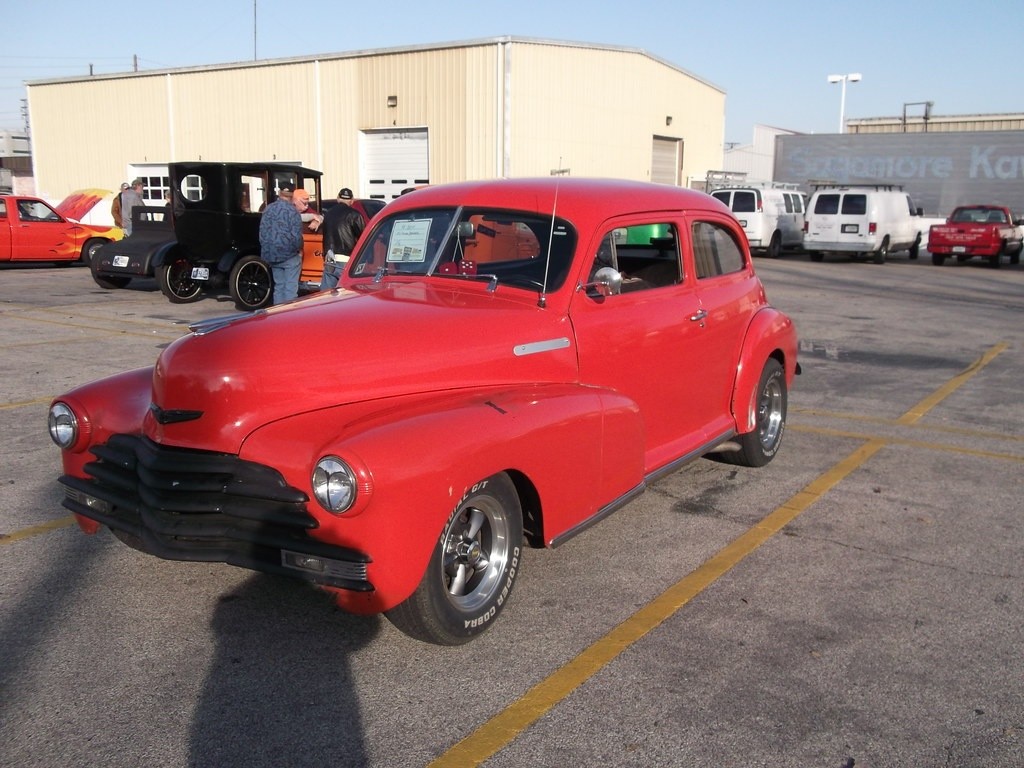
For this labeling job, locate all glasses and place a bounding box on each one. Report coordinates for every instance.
[301,201,309,205]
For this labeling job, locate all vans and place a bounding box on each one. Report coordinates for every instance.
[698,188,810,257]
[806,180,924,264]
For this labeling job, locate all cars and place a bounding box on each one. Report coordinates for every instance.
[926,204,1024,268]
[44,175,805,647]
[88,161,400,311]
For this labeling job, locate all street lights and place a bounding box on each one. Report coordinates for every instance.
[825,73,864,133]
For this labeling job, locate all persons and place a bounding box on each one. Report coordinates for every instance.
[320,188,366,292]
[110,179,171,236]
[260,182,303,305]
[292,188,325,234]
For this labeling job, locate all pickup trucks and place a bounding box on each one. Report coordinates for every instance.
[0,194,128,267]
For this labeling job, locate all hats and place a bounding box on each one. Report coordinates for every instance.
[121,183,130,189]
[292,189,309,200]
[280,182,295,192]
[132,180,146,187]
[339,188,352,199]
